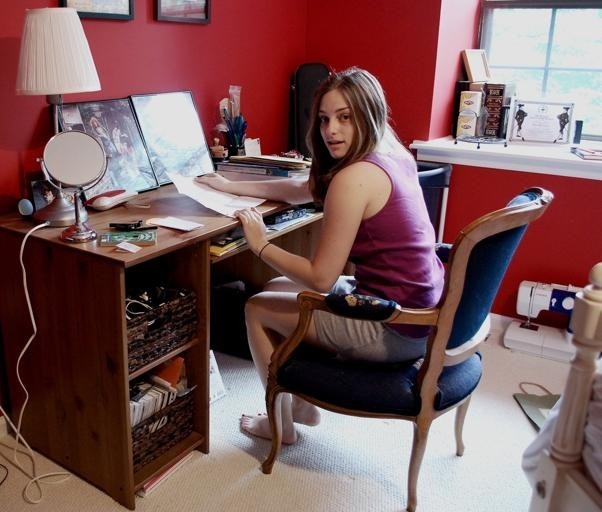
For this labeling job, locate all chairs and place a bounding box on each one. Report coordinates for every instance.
[262,185,554,511]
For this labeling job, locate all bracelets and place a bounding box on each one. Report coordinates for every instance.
[257,239,275,260]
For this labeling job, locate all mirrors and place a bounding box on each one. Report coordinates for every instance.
[40,130,109,243]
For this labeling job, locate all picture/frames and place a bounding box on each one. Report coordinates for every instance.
[504,95,576,146]
[47,98,160,205]
[460,47,492,84]
[130,89,216,185]
[153,0,211,25]
[59,0,134,23]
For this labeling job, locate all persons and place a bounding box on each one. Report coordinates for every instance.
[120,134,138,177]
[195,69,447,446]
[90,117,114,158]
[111,120,124,153]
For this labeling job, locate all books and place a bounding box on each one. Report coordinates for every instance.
[216,145,311,181]
[127,355,188,444]
[571,143,602,161]
[100,228,158,248]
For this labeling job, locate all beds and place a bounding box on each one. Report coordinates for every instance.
[526,260,601,512]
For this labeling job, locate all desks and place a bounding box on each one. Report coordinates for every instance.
[0,151,357,511]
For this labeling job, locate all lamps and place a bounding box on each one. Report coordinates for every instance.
[15,8,103,229]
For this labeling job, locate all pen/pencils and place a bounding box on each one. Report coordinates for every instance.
[223,108,247,147]
